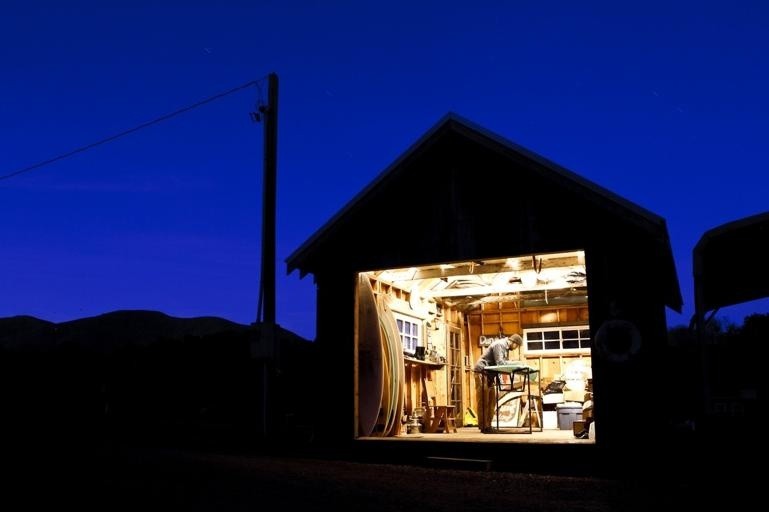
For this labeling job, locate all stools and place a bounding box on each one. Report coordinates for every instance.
[429,405,457,433]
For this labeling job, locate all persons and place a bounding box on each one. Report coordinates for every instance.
[473,333,523,433]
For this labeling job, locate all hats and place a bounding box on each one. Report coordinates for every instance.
[508,334,523,346]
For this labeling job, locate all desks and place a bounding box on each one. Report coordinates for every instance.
[477,365,544,435]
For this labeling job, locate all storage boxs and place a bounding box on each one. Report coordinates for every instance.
[555,402,584,430]
